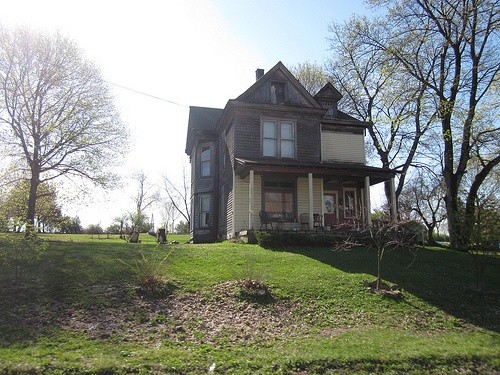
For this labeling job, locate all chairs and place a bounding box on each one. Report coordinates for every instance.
[299,213,311,230]
[259,209,273,229]
[313,214,322,231]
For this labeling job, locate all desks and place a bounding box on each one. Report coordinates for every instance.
[270,217,295,230]
